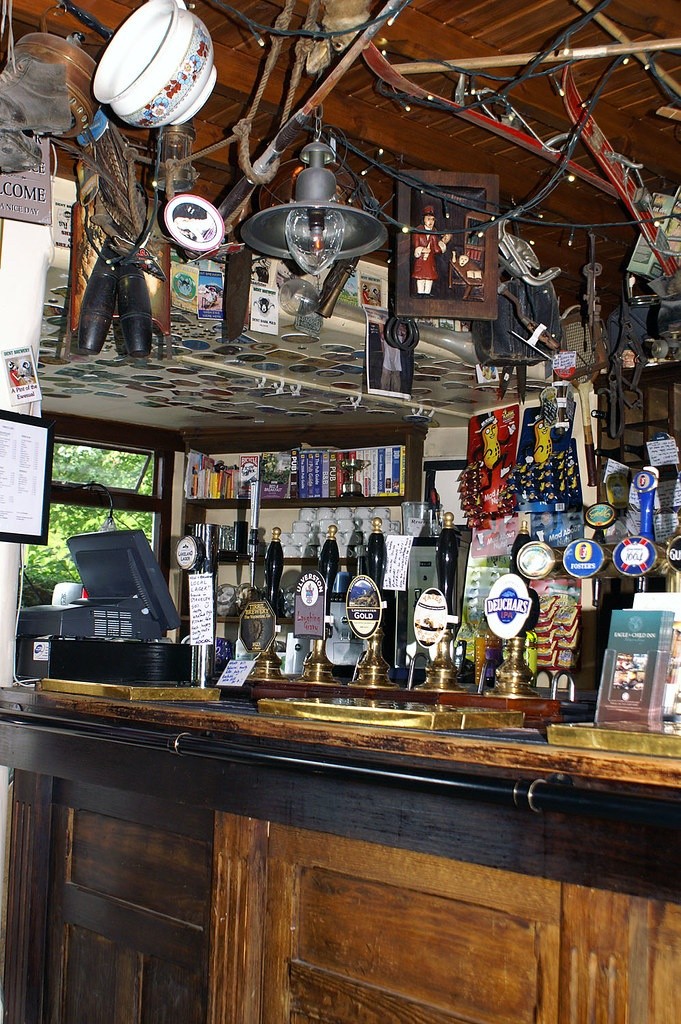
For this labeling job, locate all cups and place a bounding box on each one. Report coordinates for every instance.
[428,509,444,537]
[284,632,310,679]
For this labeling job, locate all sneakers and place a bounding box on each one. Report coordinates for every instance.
[0,131,42,173]
[0,0,72,133]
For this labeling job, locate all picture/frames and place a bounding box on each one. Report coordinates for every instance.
[398,169,500,321]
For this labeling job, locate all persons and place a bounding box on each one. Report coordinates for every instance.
[377,324,403,392]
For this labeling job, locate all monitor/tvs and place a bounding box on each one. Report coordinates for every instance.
[66,530,181,629]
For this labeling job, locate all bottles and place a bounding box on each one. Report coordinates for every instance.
[474,610,501,685]
[478,649,500,694]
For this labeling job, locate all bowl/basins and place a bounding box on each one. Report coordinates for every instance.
[93,0,217,129]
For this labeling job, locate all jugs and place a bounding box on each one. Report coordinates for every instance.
[402,502,429,537]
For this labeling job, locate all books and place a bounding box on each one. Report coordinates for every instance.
[599,608,674,728]
[184,449,250,499]
[261,447,405,498]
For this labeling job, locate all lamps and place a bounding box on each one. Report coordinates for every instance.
[240,103,388,276]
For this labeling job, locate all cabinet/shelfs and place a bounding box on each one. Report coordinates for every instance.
[178,419,427,677]
